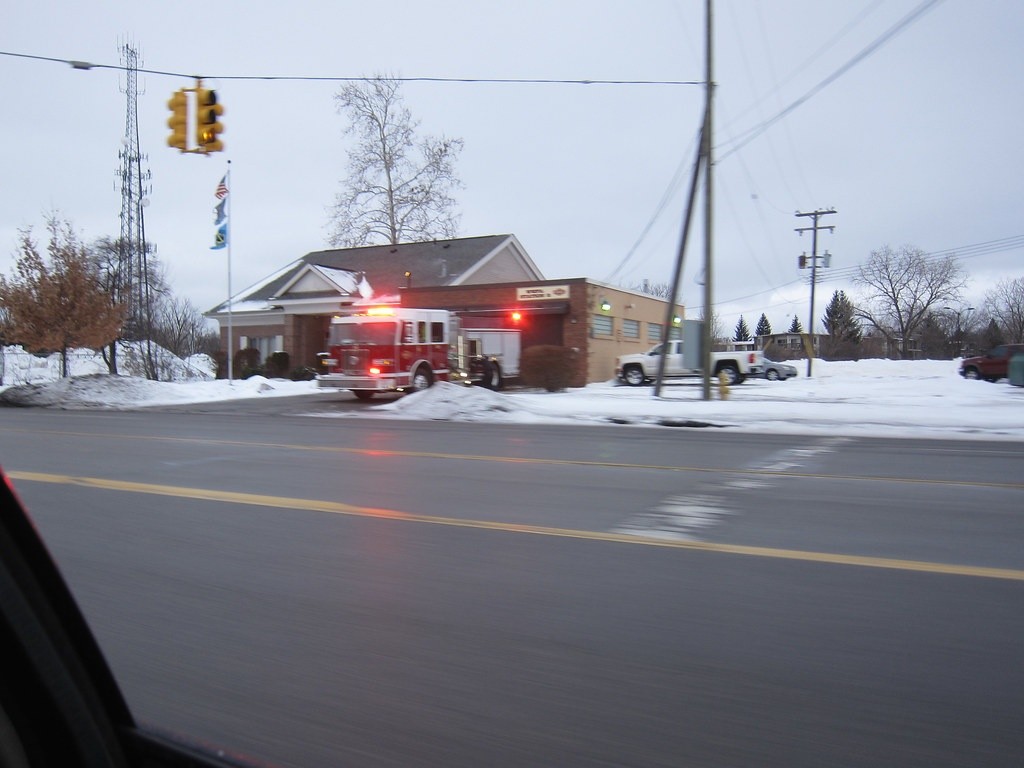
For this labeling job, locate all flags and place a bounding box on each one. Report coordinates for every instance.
[212,197,228,225]
[209,222,228,250]
[214,174,230,199]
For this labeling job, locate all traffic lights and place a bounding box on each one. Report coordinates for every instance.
[195,87,217,147]
[206,103,223,151]
[167,92,188,149]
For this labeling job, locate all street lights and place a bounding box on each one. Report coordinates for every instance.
[943,306,975,344]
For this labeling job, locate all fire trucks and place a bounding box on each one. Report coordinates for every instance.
[313,308,502,403]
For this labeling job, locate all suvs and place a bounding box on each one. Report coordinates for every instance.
[958,343,1024,382]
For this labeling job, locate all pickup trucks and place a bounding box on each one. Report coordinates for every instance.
[615,339,764,386]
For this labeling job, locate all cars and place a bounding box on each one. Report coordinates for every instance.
[745,357,798,382]
